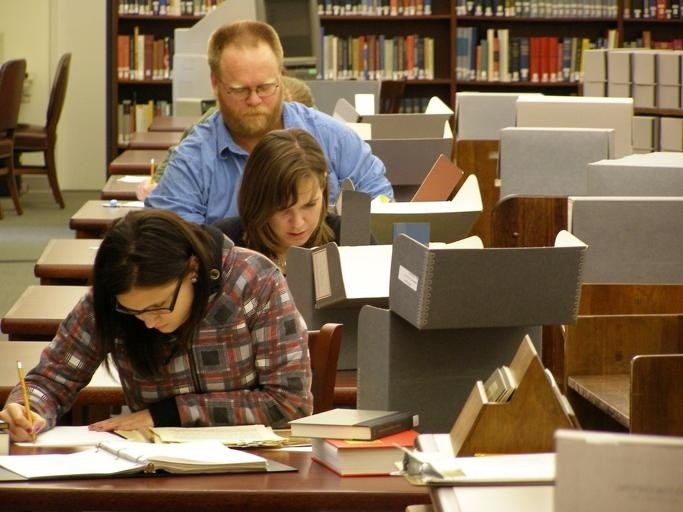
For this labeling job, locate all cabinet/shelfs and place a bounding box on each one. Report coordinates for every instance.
[106,0,224,183]
[309,0,683,142]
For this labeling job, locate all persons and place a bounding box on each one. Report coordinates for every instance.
[203,130,375,283]
[1,212,317,442]
[134,76,315,201]
[143,23,395,229]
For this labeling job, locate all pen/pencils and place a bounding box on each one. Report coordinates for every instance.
[17,361,36,444]
[151,158,154,186]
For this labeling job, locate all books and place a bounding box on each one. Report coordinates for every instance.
[318,1,434,116]
[483,367,507,403]
[455,1,619,83]
[0,442,298,482]
[312,427,424,478]
[289,407,413,441]
[620,0,682,50]
[117,0,225,147]
[500,365,516,403]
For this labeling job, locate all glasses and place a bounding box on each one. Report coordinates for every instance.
[219,80,281,104]
[113,279,184,320]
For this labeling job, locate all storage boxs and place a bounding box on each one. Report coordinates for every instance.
[454,91,540,142]
[497,126,616,198]
[585,152,683,197]
[351,221,586,439]
[567,196,683,286]
[515,96,634,160]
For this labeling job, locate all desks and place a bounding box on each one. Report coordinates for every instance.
[1,112,554,511]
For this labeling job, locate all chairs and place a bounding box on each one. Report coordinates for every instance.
[9,53,72,208]
[1,58,26,219]
